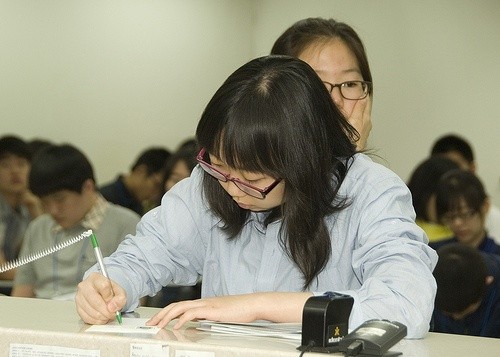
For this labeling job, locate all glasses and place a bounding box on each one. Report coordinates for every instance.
[38,189,71,210]
[196,145,283,200]
[442,198,483,223]
[322,80,372,101]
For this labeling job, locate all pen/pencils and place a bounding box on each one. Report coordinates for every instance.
[88,229,122,325]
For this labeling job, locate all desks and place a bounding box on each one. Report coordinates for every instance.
[0,295,500,357]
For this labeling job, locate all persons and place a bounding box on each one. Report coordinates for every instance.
[0,134,203,299]
[407,134,500,339]
[270,17,372,150]
[76,57,438,339]
